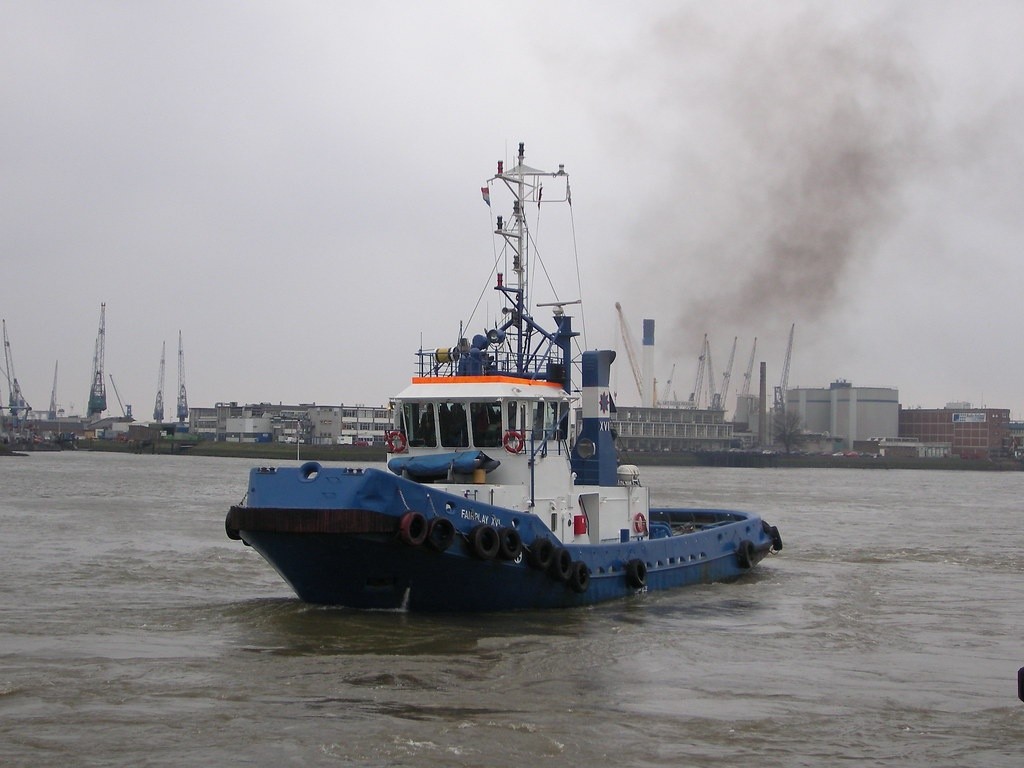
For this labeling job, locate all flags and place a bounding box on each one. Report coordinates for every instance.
[482,187,491,206]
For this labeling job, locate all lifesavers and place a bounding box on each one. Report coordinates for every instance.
[386,431,406,453]
[738,539,757,568]
[627,558,648,589]
[636,513,647,531]
[502,430,525,454]
[552,547,571,579]
[223,506,251,548]
[399,513,455,552]
[761,519,783,552]
[534,537,556,570]
[571,560,591,593]
[469,524,524,562]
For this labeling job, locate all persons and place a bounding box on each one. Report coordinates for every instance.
[401,402,501,447]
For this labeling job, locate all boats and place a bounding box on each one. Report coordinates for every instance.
[222,140,785,614]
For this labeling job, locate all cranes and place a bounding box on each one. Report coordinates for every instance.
[0,301,191,441]
[613,302,796,450]
